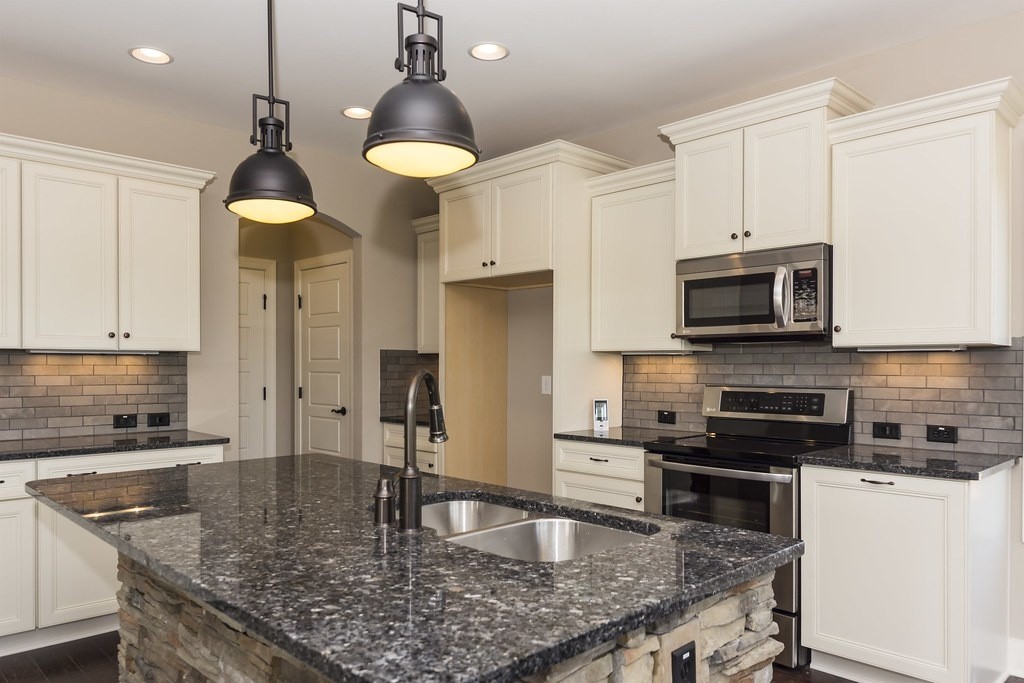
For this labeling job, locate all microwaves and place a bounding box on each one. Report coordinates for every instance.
[675,243,831,342]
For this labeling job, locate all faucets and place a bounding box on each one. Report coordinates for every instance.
[400,370,449,538]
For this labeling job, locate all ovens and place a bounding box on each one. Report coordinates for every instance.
[643,452,809,668]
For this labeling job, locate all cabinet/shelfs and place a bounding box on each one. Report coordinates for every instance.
[0,445,227,657]
[0,134,217,355]
[554,439,644,511]
[411,217,442,353]
[799,465,1023,683]
[590,181,712,355]
[438,162,553,283]
[380,425,438,477]
[832,111,1024,348]
[675,107,832,262]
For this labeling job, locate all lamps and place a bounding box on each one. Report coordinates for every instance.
[222,0,318,224]
[363,0,483,179]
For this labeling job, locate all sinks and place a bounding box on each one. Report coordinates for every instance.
[445,518,661,563]
[395,499,530,537]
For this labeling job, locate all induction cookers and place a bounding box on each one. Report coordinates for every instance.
[644,385,854,467]
